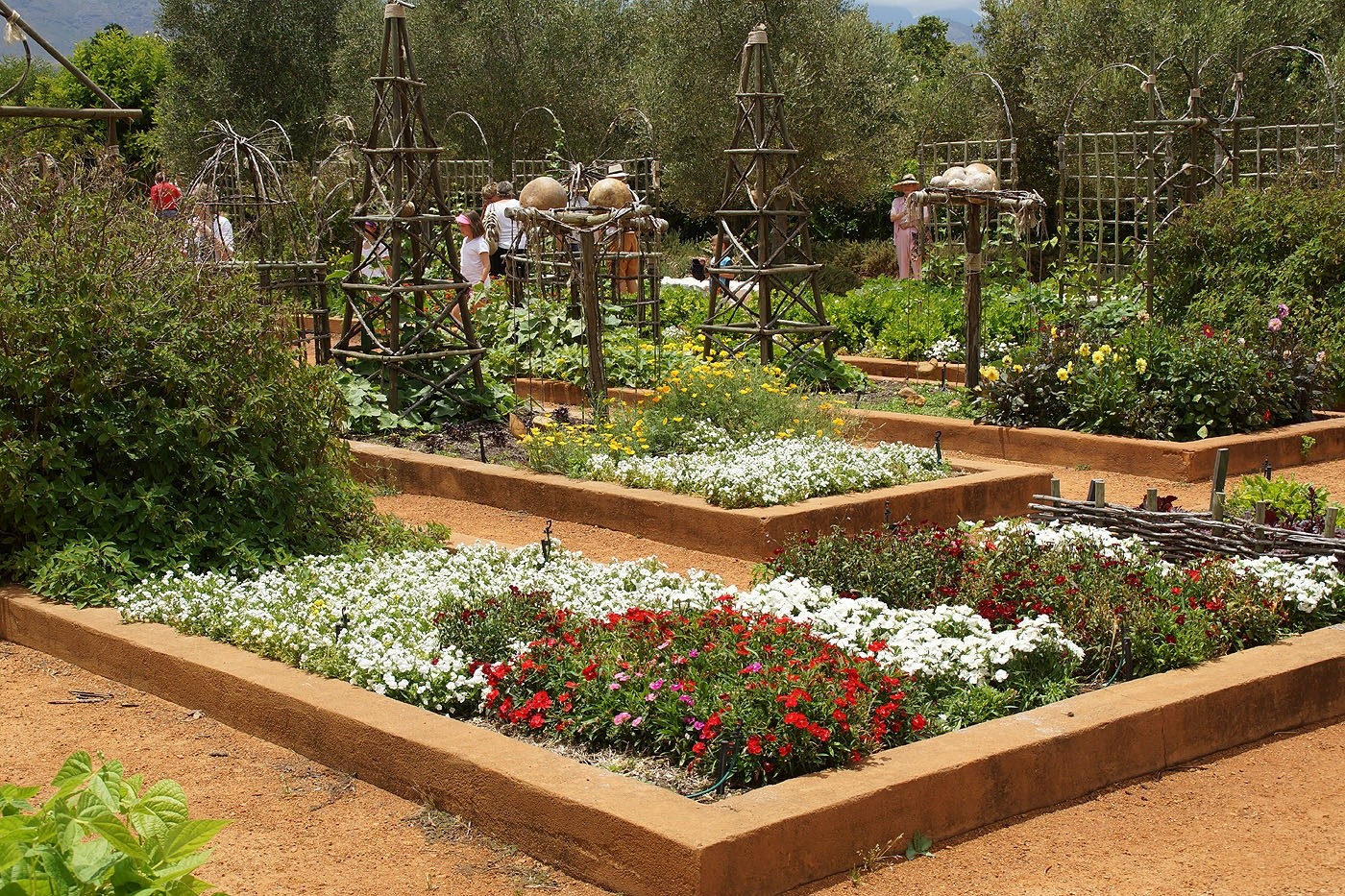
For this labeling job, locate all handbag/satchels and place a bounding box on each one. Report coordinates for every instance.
[482,203,500,254]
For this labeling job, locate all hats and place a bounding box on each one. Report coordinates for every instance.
[456,214,471,224]
[606,164,629,177]
[892,174,920,191]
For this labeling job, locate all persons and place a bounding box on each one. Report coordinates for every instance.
[356,220,391,314]
[889,173,930,281]
[148,170,234,277]
[692,235,737,302]
[452,163,640,325]
